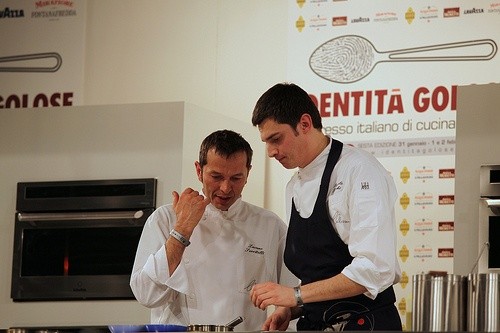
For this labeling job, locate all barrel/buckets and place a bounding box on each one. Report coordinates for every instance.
[467,273,500,333]
[411,274,467,333]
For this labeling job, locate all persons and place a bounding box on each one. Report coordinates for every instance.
[249,85,403,331]
[130,129,287,331]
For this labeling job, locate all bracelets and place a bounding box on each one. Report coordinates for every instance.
[294,286,304,308]
[169,229,191,247]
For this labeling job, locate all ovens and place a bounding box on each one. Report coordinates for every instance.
[12,178,157,299]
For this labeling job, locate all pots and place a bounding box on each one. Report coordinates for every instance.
[186,316,245,333]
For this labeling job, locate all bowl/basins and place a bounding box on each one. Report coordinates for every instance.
[108,325,145,333]
[146,325,185,333]
